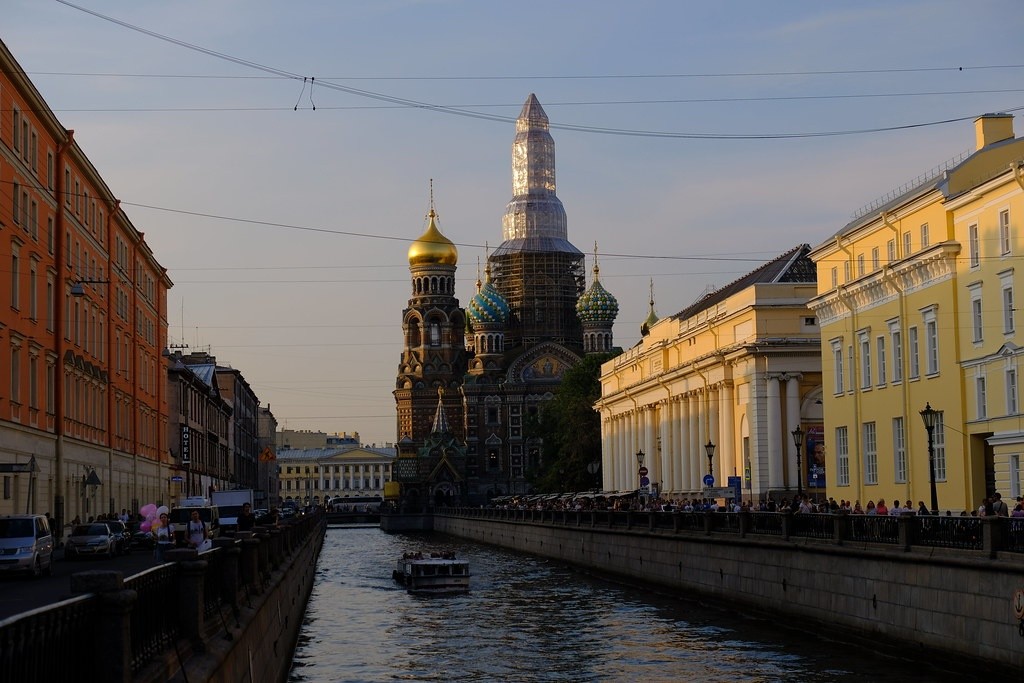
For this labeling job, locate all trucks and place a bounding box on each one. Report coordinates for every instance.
[213,489,254,534]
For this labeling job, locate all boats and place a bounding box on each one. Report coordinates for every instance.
[393,550,471,597]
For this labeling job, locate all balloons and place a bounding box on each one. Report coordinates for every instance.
[139,504,169,534]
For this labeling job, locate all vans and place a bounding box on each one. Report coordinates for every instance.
[168,495,219,545]
[0,512,53,581]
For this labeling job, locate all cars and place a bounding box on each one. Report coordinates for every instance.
[252,514,286,533]
[64,522,116,560]
[101,520,131,554]
[283,508,295,517]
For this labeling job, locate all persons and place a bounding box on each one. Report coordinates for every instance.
[402,549,457,561]
[236,502,256,532]
[264,491,1023,550]
[808,442,825,471]
[5,508,132,551]
[183,508,209,550]
[152,513,177,566]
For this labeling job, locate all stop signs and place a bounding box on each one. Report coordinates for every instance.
[639,467,648,476]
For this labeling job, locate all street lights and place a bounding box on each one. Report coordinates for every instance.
[791,425,806,495]
[920,401,941,516]
[71,278,115,522]
[636,449,645,488]
[704,440,717,506]
